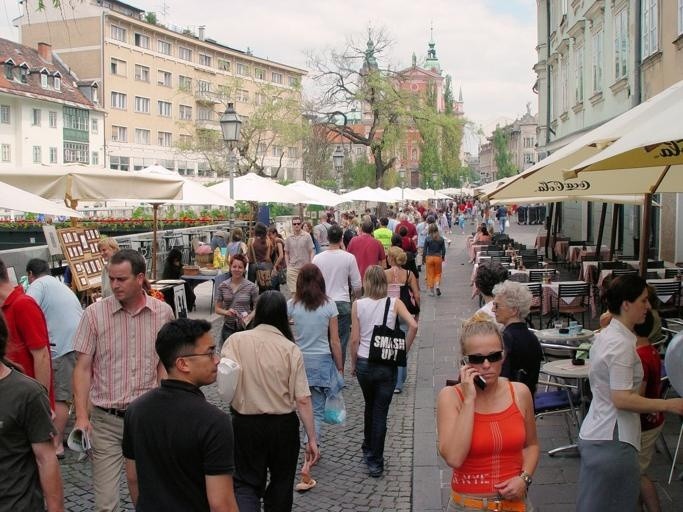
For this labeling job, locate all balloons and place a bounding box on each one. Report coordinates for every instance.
[666,328,682,397]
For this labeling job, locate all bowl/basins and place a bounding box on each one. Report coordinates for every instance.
[182,247,223,276]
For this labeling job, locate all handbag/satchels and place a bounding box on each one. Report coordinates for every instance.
[237,311,252,329]
[399,270,420,315]
[367,297,407,368]
[255,269,273,288]
[278,269,287,285]
[406,238,418,261]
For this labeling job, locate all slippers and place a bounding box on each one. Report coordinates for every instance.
[295,477,317,491]
[313,446,321,466]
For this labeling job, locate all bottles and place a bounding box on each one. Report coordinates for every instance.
[506,244,523,271]
[542,272,551,284]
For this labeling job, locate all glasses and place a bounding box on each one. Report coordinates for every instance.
[292,223,301,225]
[492,301,509,310]
[177,350,218,359]
[463,350,504,363]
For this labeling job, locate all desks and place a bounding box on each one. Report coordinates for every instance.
[136,275,191,332]
[174,266,236,315]
[517,319,681,489]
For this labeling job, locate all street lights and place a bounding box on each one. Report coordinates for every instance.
[219,99,240,244]
[398,162,404,210]
[332,145,345,227]
[431,170,436,204]
[458,175,462,205]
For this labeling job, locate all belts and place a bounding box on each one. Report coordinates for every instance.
[451,490,526,512]
[96,406,125,418]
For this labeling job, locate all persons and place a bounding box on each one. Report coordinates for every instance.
[0,259,56,448]
[0,316,66,512]
[286,263,344,487]
[630,309,665,511]
[597,275,660,354]
[435,319,541,511]
[72,249,176,511]
[464,262,510,363]
[573,274,682,511]
[120,318,241,511]
[492,280,543,424]
[0,198,475,365]
[380,246,420,394]
[216,289,319,511]
[346,265,418,477]
[24,259,82,461]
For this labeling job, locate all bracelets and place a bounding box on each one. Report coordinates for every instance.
[521,470,531,485]
[336,367,343,373]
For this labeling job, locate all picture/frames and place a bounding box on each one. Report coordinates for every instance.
[59,228,106,287]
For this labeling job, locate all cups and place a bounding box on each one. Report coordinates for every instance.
[554,319,584,336]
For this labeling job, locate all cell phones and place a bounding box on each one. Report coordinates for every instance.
[461,360,486,391]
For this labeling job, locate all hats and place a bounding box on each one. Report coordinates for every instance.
[213,230,225,238]
[254,222,266,231]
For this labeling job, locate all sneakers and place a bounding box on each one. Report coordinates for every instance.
[436,288,441,296]
[428,293,433,296]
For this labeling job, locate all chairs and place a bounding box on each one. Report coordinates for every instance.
[113,225,242,275]
[468,228,683,330]
